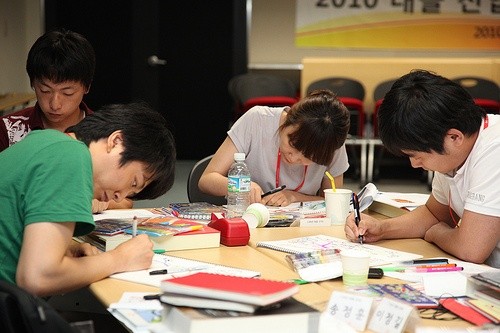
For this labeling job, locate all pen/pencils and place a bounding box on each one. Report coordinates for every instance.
[133,216,137,237]
[149,266,207,275]
[150,207,176,217]
[353,193,363,244]
[153,249,167,253]
[400,259,464,272]
[261,185,286,198]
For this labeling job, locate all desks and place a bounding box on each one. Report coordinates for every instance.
[74,195,500,333]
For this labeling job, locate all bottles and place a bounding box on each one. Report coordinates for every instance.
[227,153,251,219]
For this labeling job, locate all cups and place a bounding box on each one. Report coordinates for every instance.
[340,249,374,286]
[242,203,270,229]
[323,188,352,226]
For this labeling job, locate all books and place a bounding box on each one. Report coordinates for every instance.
[169,202,223,220]
[81,216,221,252]
[160,272,299,313]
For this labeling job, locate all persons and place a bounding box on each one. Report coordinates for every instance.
[0,30,95,151]
[0,103,176,301]
[344,69,500,269]
[199,90,350,207]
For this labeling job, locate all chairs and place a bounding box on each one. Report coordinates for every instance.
[303,76,366,186]
[368,76,432,191]
[187,154,227,207]
[0,279,78,333]
[452,72,500,113]
[227,75,299,110]
